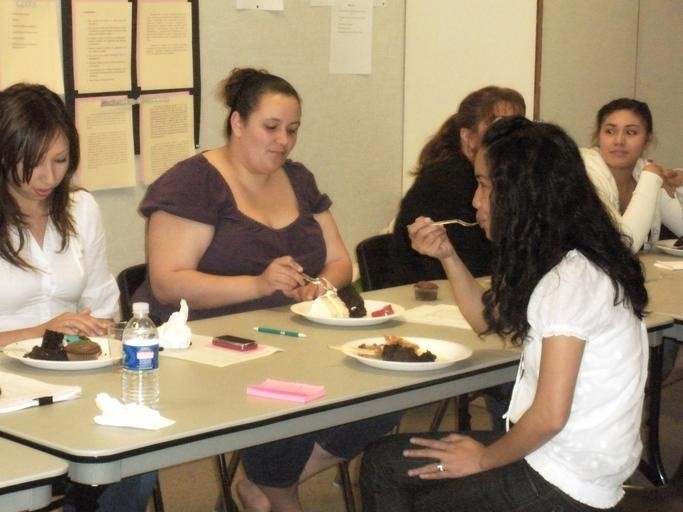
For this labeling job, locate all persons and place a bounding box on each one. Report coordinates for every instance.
[388,84,526,432]
[0,82,158,511]
[138,66,410,512]
[575,97,682,258]
[359,115,647,512]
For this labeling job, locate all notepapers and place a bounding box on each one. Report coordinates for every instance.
[247,378,326,404]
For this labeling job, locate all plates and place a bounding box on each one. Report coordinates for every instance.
[290,295,470,370]
[655,237,680,259]
[2,333,122,370]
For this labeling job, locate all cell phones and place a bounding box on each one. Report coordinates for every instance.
[213,335,258,352]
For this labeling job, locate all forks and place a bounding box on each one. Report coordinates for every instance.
[408,217,479,229]
[295,265,339,295]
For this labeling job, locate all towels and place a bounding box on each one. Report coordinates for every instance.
[393,302,472,330]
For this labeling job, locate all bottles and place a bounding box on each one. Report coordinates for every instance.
[121,302,161,416]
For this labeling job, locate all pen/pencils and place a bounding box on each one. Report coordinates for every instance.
[252,326,306,338]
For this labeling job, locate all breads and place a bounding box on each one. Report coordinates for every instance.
[64,339,101,361]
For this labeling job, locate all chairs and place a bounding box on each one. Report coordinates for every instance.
[116,264,357,512]
[356,233,486,431]
[608,456,683,511]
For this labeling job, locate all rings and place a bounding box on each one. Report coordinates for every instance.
[437,464,445,471]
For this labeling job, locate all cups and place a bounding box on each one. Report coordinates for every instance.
[105,321,132,373]
[414,281,438,301]
